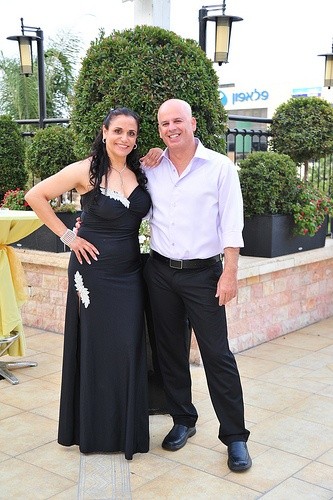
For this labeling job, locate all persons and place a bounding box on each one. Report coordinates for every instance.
[23,107,163,454]
[74,98,252,473]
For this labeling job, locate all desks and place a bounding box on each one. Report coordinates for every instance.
[0,210,45,384]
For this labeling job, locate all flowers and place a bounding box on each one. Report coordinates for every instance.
[1,188,80,214]
[239,149,333,237]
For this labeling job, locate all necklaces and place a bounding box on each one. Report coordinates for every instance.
[108,164,126,186]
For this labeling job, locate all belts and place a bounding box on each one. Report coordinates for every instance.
[149,248,223,270]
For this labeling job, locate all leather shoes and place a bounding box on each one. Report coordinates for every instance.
[161,424,196,451]
[227,441,252,473]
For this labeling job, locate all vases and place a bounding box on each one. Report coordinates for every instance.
[241,213,330,257]
[9,211,81,253]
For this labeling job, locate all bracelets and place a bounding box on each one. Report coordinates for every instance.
[59,230,77,245]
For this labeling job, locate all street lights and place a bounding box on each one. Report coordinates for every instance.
[198,0,244,66]
[6,18,47,119]
